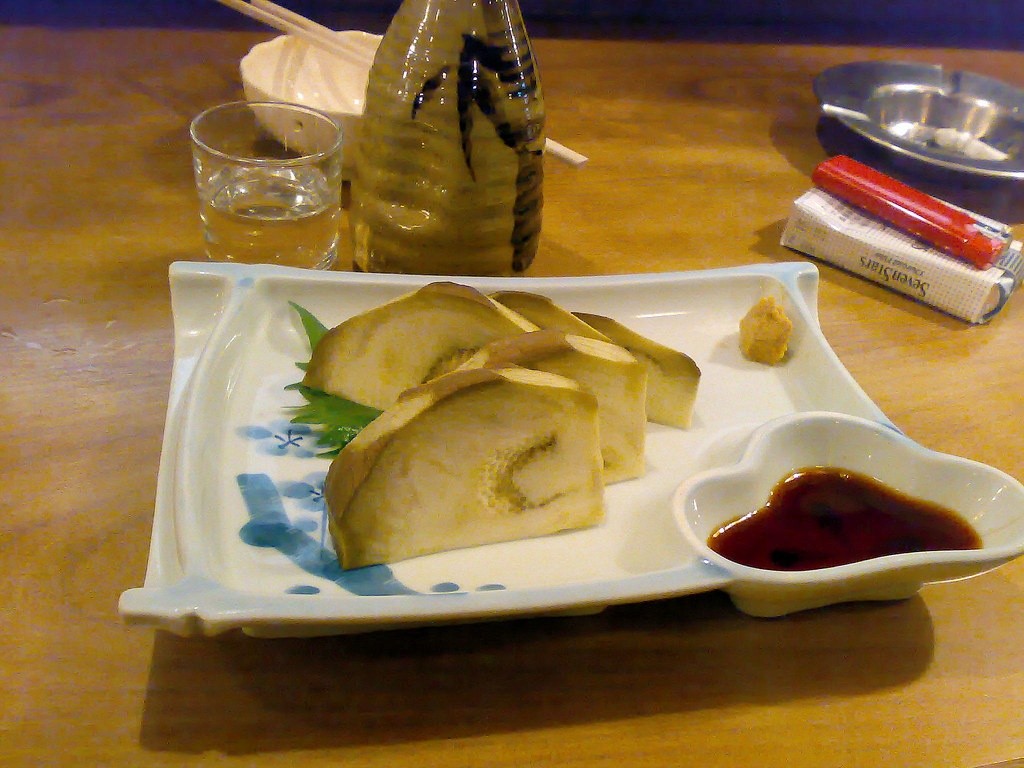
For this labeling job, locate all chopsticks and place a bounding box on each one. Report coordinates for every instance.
[212,0,590,171]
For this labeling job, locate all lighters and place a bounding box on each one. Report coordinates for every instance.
[810,153,1014,271]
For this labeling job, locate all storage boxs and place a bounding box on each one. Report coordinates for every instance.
[779,186,1024,325]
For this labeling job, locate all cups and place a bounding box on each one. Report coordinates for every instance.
[188,101,344,269]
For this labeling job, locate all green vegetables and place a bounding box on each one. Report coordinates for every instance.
[286,300,386,462]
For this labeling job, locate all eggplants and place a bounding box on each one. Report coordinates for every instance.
[300,278,701,571]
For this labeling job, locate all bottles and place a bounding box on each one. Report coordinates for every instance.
[349,0,546,276]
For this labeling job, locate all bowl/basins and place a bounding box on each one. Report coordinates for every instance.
[240,31,385,182]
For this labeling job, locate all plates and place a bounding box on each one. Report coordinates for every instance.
[813,59,1024,189]
[117,257,1024,641]
